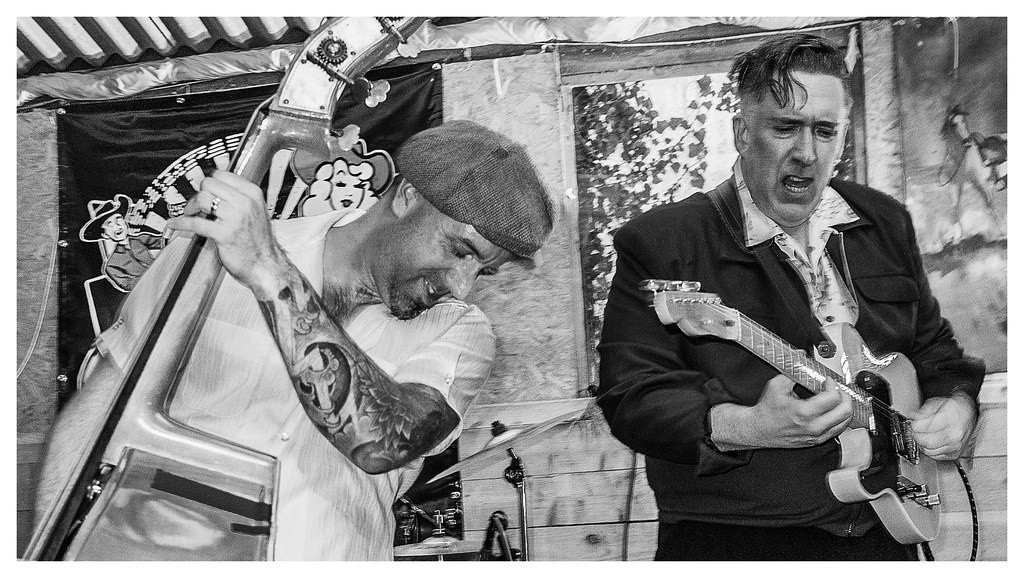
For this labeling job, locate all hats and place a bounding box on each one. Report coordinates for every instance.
[396,120,555,258]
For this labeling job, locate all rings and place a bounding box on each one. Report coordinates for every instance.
[208,197,220,218]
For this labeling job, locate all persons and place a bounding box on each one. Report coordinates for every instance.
[599,35,986,560]
[28,119,555,561]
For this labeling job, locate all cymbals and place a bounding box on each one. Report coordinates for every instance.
[394,537,486,556]
[425,407,590,483]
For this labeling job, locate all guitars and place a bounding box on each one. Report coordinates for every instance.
[18,16,425,559]
[646,277,939,545]
